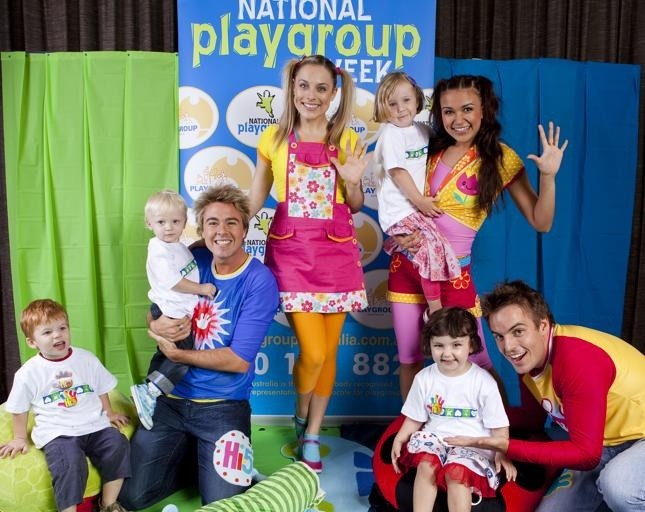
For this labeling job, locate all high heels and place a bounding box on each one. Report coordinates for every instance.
[298,434,322,473]
[295,416,308,438]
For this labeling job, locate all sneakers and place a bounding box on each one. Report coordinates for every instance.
[130,384,156,430]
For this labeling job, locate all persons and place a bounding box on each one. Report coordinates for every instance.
[0,299,133,512]
[375,72,463,325]
[390,306,519,512]
[116,183,272,511]
[444,280,645,512]
[129,191,216,431]
[387,74,570,432]
[186,54,374,475]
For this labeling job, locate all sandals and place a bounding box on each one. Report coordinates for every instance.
[97,496,133,512]
